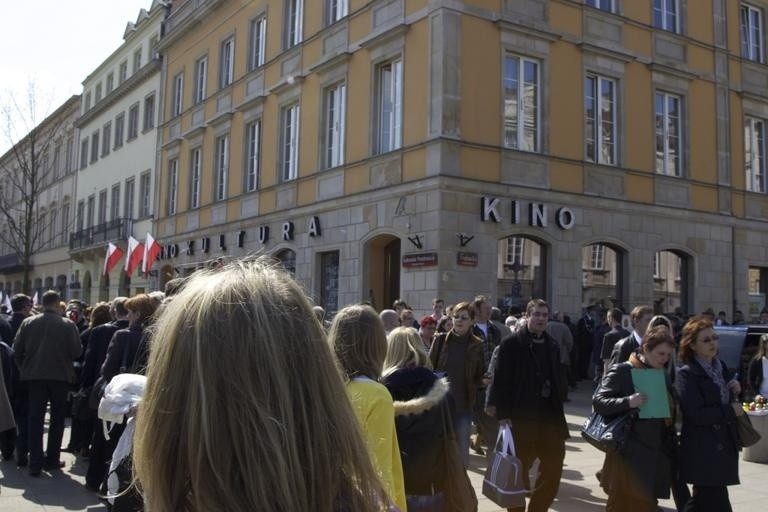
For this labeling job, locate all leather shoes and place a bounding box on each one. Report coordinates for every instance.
[45,459,65,470]
[471,440,484,455]
[29,463,40,475]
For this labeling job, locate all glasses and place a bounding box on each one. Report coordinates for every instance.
[452,313,469,320]
[695,334,718,343]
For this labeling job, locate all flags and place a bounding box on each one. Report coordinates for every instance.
[103,241,124,275]
[142,232,160,273]
[125,236,144,278]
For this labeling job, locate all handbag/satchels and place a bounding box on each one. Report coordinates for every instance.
[737,410,762,446]
[483,423,526,509]
[88,328,129,410]
[582,365,639,454]
[439,394,478,512]
[70,385,95,421]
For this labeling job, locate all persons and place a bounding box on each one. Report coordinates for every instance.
[86,293,150,492]
[473,295,502,454]
[380,309,398,334]
[485,299,571,511]
[24,295,34,317]
[61,305,111,454]
[10,294,28,328]
[577,305,594,376]
[629,316,691,511]
[313,305,331,328]
[594,308,611,385]
[326,305,408,511]
[165,278,186,296]
[150,291,165,304]
[109,297,117,323]
[60,301,66,317]
[719,311,729,325]
[546,311,574,400]
[430,298,445,322]
[600,307,632,375]
[436,316,453,332]
[382,327,456,512]
[759,309,768,323]
[675,317,744,512]
[612,305,654,364]
[748,335,768,401]
[0,304,12,315]
[447,304,455,317]
[394,299,420,329]
[8,294,29,324]
[426,301,489,468]
[132,256,399,512]
[489,306,527,336]
[67,300,90,336]
[81,296,129,396]
[13,290,83,479]
[419,316,438,356]
[593,326,675,512]
[400,309,414,327]
[732,310,745,324]
[564,316,577,370]
[0,314,28,467]
